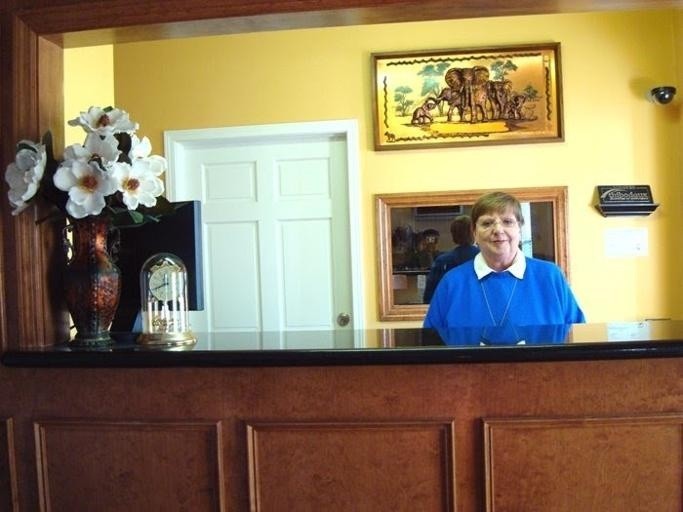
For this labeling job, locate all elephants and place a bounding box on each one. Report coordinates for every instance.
[436,65,526,123]
[411,97,436,124]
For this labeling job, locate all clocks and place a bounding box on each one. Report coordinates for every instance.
[147,260,186,304]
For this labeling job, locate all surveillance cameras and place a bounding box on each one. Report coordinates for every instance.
[651,86,676,104]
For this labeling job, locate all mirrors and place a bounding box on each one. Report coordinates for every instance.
[374,184,571,323]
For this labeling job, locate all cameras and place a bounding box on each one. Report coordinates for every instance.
[426,237,435,244]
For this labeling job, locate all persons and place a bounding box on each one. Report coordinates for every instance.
[407,228,444,268]
[424,192,586,325]
[423,215,481,303]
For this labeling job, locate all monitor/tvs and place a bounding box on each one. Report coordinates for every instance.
[413,207,464,217]
[107,200,204,343]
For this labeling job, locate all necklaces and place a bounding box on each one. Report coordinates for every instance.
[479,277,518,326]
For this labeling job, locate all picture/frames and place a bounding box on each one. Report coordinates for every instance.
[369,41,564,150]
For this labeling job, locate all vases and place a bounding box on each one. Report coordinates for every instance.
[5,105,170,226]
[57,218,121,347]
